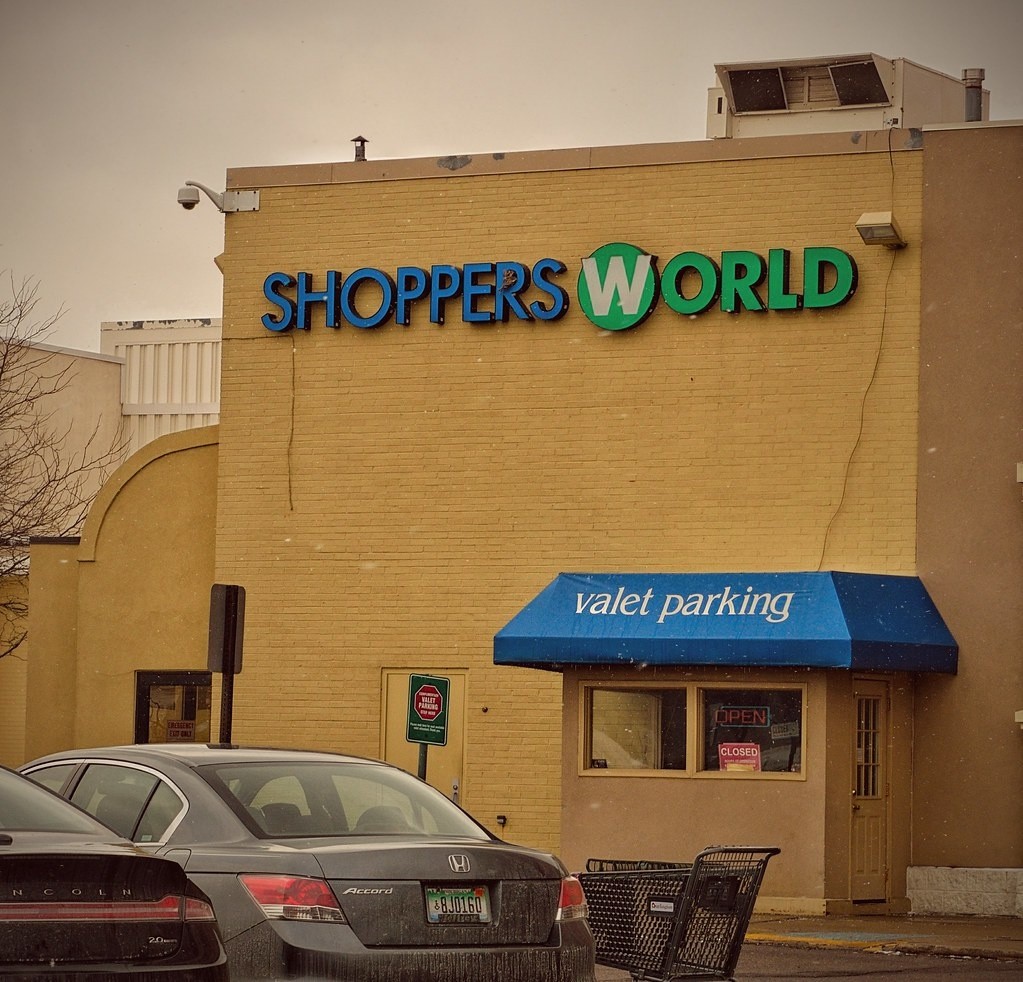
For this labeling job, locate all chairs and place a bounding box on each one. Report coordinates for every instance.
[356,806,409,831]
[94,794,153,842]
[261,803,331,835]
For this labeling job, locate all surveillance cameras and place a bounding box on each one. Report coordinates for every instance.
[177,186,200,209]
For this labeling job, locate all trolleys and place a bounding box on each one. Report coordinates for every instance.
[568,843,781,982]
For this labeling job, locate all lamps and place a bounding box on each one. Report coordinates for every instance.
[855,212,909,249]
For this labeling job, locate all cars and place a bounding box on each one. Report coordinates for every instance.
[0,766,231,982]
[8,741,597,982]
